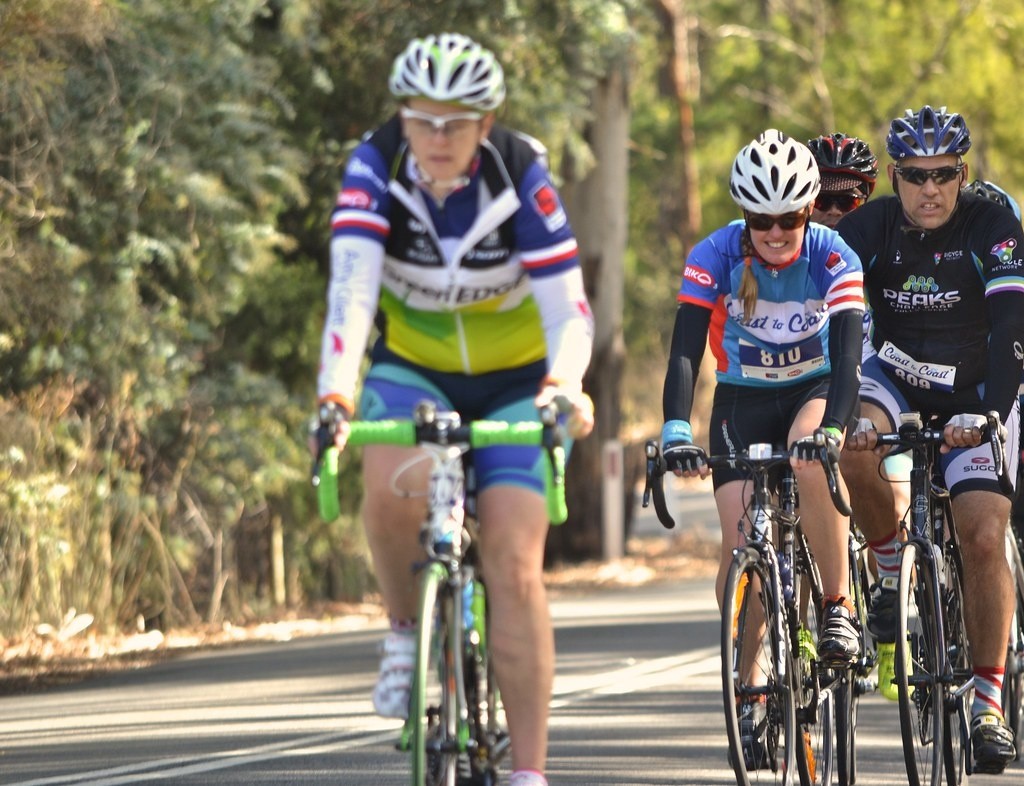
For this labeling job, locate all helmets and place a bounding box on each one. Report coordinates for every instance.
[887,105,972,160]
[388,32,506,111]
[807,132,880,198]
[729,128,821,215]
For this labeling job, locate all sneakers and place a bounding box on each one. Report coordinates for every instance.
[869,583,901,643]
[877,631,914,701]
[817,595,862,658]
[373,630,418,719]
[797,622,818,663]
[970,711,1017,774]
[726,700,771,772]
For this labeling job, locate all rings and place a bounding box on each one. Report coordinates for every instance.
[963,429,971,434]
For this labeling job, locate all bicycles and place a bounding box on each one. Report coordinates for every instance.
[308,398,577,786]
[818,410,1024,786]
[641,437,881,786]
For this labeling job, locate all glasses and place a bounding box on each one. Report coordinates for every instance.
[745,213,808,232]
[401,104,485,139]
[895,162,965,186]
[814,193,867,213]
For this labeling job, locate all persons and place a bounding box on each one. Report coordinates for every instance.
[792,134,913,700]
[661,126,867,772]
[307,28,595,786]
[832,106,1024,767]
